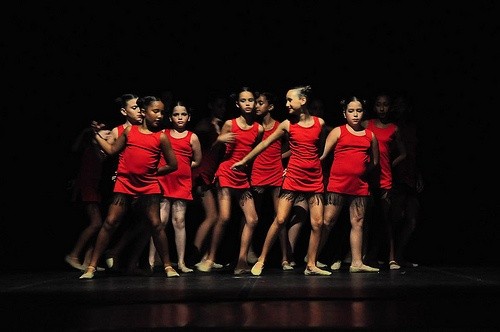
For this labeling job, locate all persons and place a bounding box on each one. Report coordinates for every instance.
[305,93,425,273]
[65,92,203,280]
[191,84,333,277]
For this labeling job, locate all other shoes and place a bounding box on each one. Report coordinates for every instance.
[304,267,332,276]
[234,268,250,275]
[389,260,401,269]
[197,260,214,272]
[330,260,341,270]
[251,262,264,275]
[164,266,180,277]
[247,256,258,263]
[80,264,105,271]
[349,264,380,273]
[64,256,86,270]
[282,261,293,270]
[304,257,328,267]
[201,260,223,268]
[401,263,418,267]
[80,265,95,278]
[178,263,193,272]
